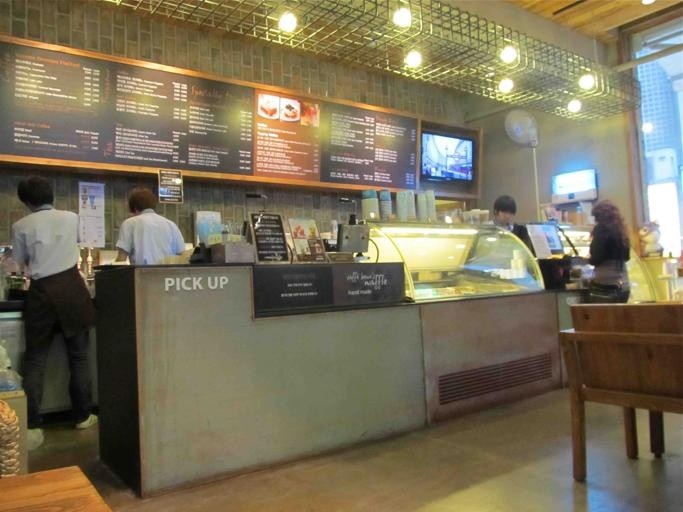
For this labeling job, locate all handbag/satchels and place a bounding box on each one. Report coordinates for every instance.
[584,279,624,303]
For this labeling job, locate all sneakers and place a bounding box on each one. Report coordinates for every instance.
[75,414,98,429]
[28,428,44,436]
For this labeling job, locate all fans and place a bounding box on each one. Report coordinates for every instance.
[505,109,539,147]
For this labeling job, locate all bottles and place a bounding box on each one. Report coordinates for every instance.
[576,205,586,227]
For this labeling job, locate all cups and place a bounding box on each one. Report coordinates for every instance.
[462,209,491,227]
[379,187,437,222]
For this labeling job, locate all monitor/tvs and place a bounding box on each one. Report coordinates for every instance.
[420,130,475,186]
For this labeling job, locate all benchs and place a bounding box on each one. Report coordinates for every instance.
[557,303,683,482]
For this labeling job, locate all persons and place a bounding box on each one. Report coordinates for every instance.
[483,194,534,254]
[583,197,632,304]
[113,185,185,267]
[10,175,99,451]
